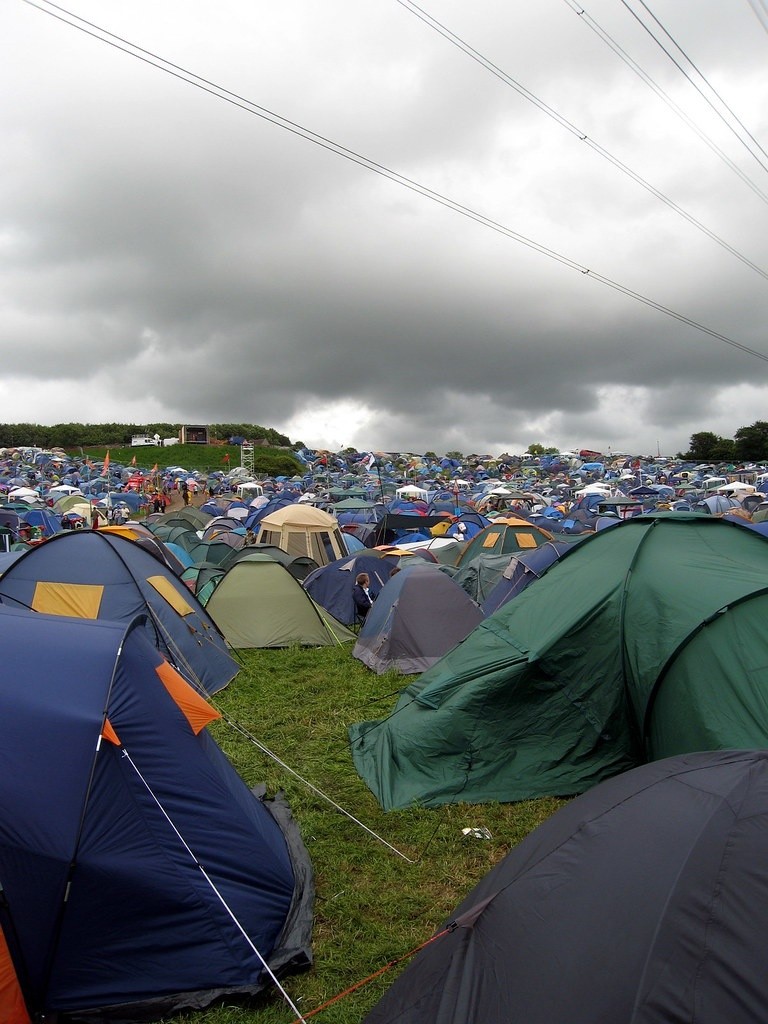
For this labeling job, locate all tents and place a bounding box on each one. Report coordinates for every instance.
[0,436,768,1024]
[350,512,768,812]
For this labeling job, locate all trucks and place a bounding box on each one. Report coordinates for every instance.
[132,434,158,447]
[163,438,179,447]
[230,436,248,446]
